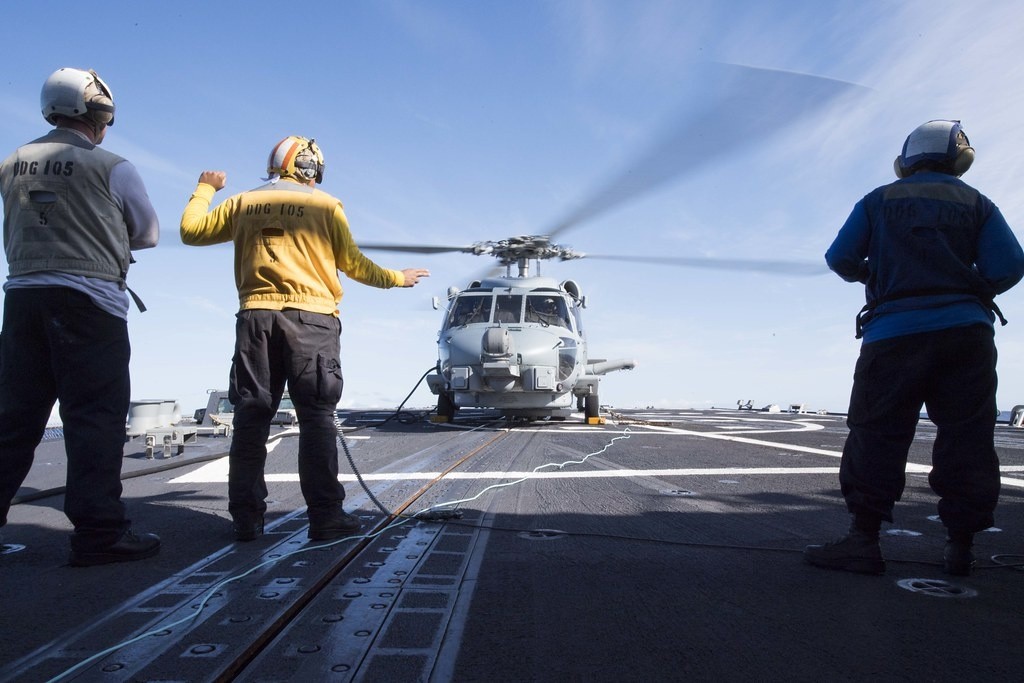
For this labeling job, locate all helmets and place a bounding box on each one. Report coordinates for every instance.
[895,118,974,175]
[267,135,325,185]
[40,67,115,126]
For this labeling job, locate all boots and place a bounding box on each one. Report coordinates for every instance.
[802,513,887,573]
[943,527,976,569]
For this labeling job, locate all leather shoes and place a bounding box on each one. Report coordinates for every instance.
[232,517,264,538]
[307,500,361,539]
[70,529,162,565]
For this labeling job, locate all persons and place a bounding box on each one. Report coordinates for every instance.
[540,298,558,315]
[802,118,1024,579]
[181,134,431,543]
[1,67,163,566]
[471,302,481,313]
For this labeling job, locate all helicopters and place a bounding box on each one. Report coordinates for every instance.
[350,66,867,426]
